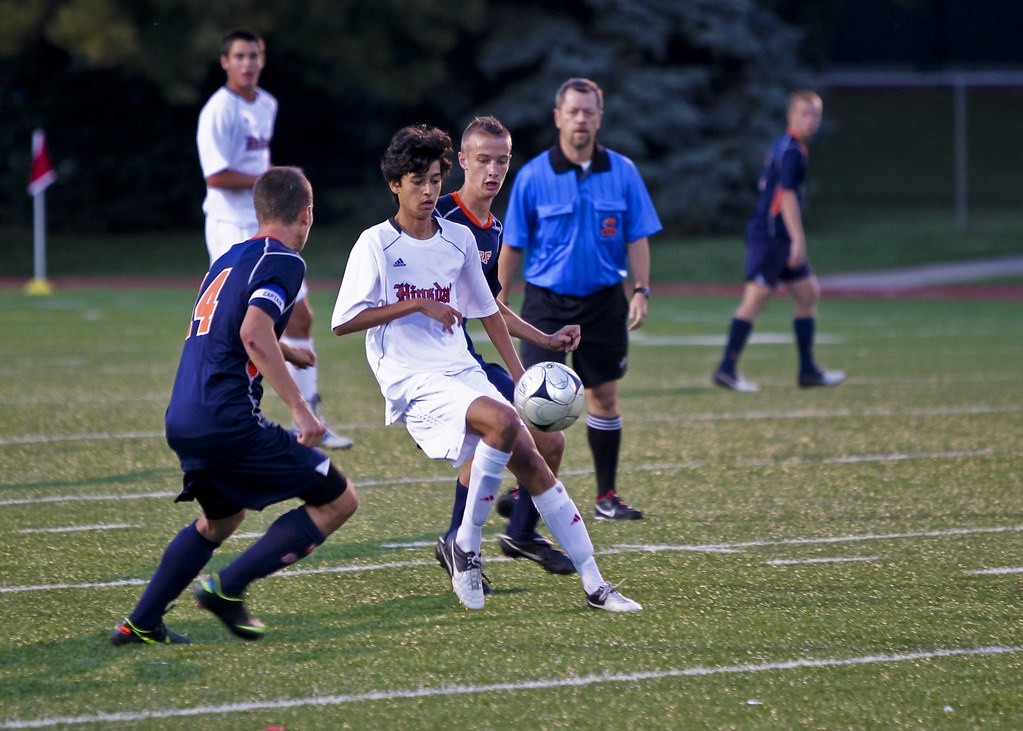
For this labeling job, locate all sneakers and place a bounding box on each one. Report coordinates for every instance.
[446,530,485,610]
[499,524,578,574]
[110,604,191,646]
[436,535,492,595]
[583,577,644,613]
[594,489,642,520]
[799,370,846,387]
[714,369,759,393]
[496,484,519,518]
[292,419,355,450]
[192,572,267,641]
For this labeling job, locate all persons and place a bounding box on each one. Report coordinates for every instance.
[194,26,353,452]
[423,115,581,578]
[496,77,663,520]
[108,164,359,646]
[711,94,847,393]
[331,125,642,615]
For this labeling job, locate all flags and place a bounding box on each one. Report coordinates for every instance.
[24,129,58,197]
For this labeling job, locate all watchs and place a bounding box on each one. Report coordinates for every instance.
[634,287,651,299]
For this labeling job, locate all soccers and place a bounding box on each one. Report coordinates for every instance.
[514,362,585,433]
